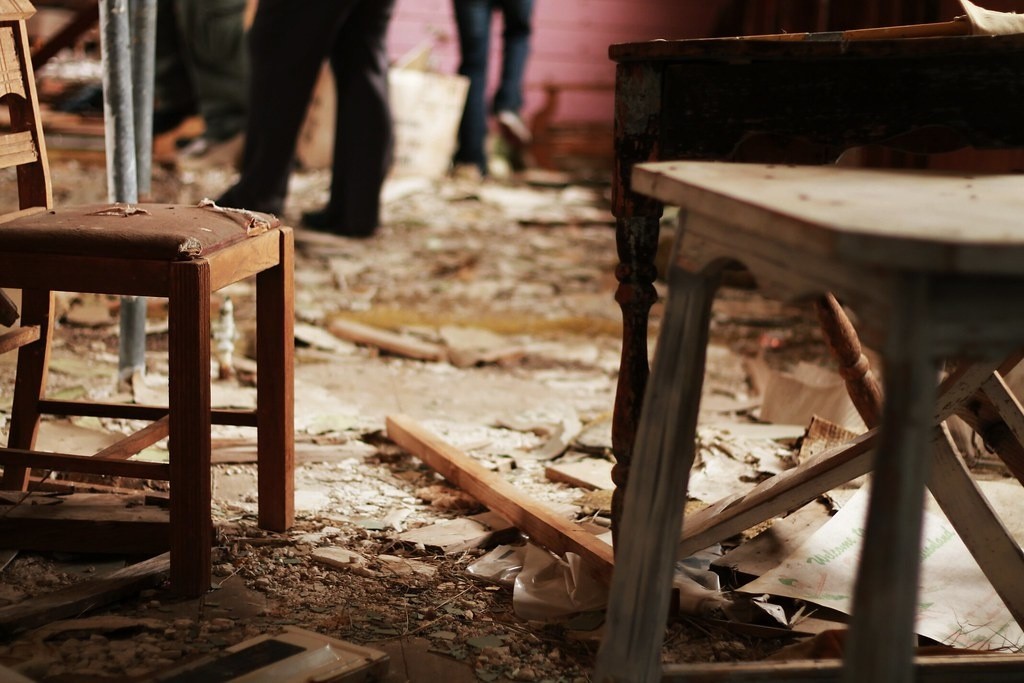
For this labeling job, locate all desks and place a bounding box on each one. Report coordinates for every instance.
[593,160,1024,683]
[610,32,1024,568]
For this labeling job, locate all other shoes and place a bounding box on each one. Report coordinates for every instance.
[498,109,532,148]
[213,186,286,220]
[438,163,484,201]
[300,202,378,239]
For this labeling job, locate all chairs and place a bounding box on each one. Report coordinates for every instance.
[0,0,295,601]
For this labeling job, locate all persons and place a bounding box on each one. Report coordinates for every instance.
[213,0,402,243]
[453,0,534,185]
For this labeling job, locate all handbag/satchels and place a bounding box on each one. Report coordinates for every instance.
[297,27,470,176]
